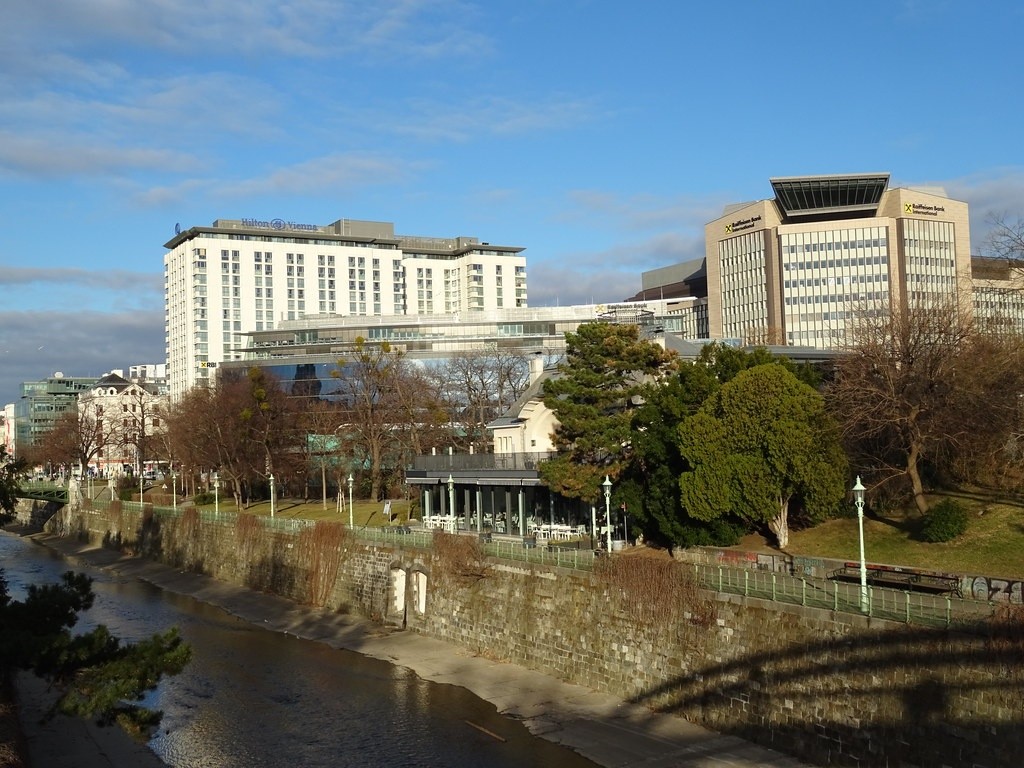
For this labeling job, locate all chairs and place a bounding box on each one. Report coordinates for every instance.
[421,512,586,541]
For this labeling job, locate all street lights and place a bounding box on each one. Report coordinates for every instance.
[139,474,143,507]
[447,473,454,534]
[347,473,354,530]
[852,475,870,613]
[269,473,275,518]
[171,474,177,510]
[214,479,220,516]
[602,474,612,557]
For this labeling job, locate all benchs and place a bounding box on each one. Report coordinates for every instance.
[833,561,965,603]
